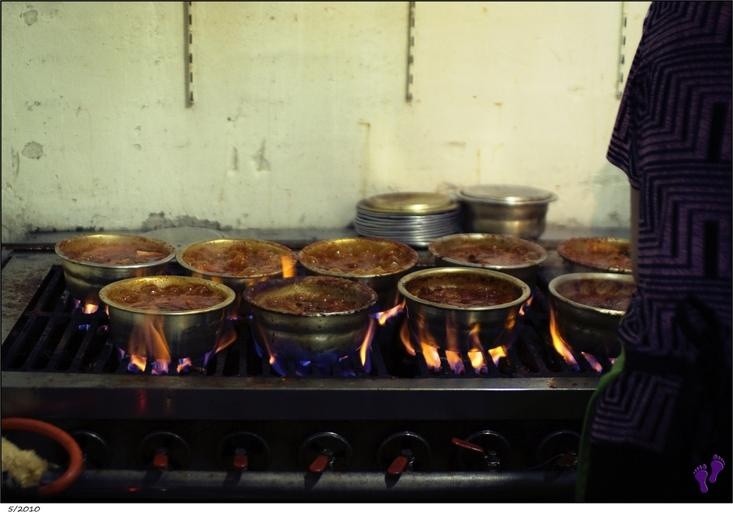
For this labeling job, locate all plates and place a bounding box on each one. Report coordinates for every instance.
[457,184,559,205]
[352,189,459,248]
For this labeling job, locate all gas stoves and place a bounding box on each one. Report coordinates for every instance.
[2,260,624,377]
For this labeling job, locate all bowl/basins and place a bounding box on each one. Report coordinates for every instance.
[53,231,656,359]
[463,203,548,241]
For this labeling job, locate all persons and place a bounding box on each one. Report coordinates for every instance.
[581,1,732,503]
